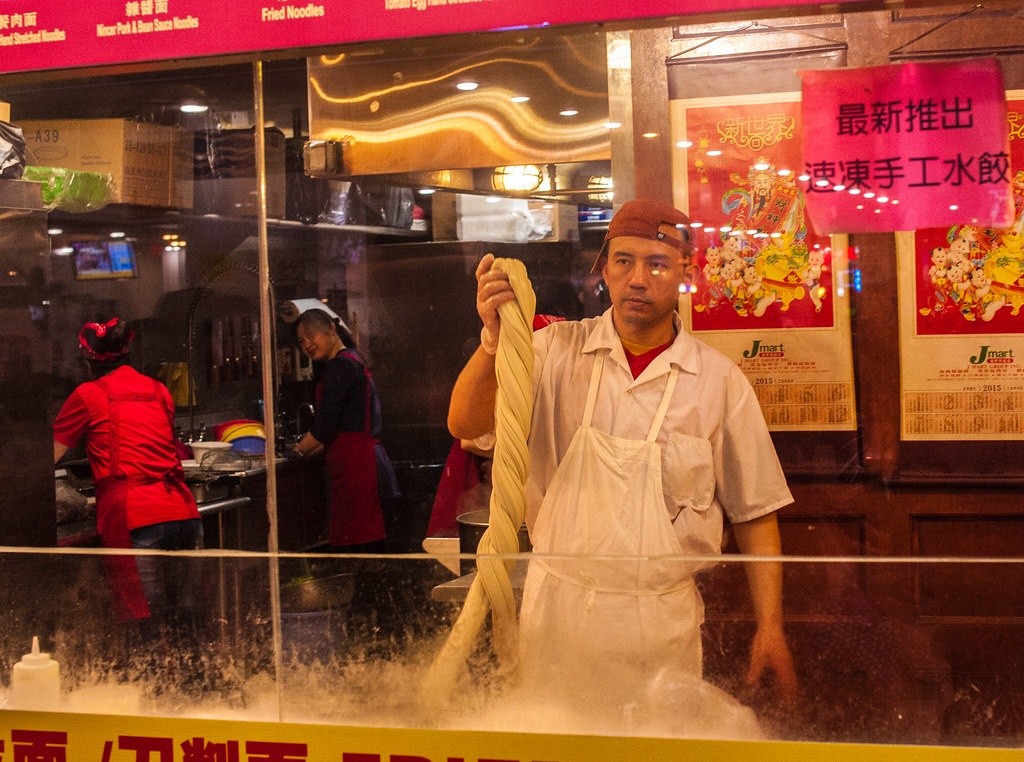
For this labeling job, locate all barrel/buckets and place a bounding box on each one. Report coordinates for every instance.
[272,605,351,661]
[456,507,533,578]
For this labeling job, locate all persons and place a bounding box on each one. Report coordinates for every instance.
[288,310,386,625]
[448,198,799,703]
[54,318,204,691]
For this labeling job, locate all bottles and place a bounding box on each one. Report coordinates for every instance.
[11,635,60,712]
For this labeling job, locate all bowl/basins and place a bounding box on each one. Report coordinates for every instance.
[190,441,234,463]
[215,419,266,455]
[264,572,355,614]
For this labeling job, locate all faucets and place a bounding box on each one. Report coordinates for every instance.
[188,387,209,444]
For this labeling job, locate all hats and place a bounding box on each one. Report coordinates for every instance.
[589,199,694,274]
[77,318,134,360]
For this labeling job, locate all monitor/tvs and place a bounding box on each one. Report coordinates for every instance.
[68,237,140,280]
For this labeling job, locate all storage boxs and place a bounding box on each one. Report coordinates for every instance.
[3,119,197,211]
[527,202,580,243]
